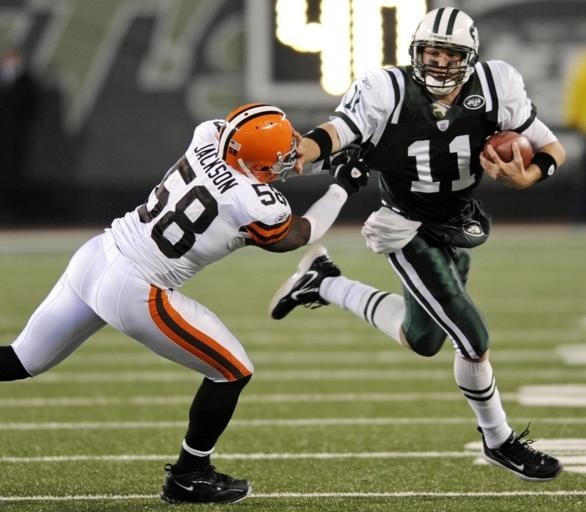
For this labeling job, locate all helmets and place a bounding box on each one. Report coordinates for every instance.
[217,102,297,185]
[409,7,480,84]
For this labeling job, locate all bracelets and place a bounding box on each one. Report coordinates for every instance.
[530,151,558,182]
[302,127,333,163]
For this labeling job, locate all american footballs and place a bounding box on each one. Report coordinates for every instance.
[481,131,532,169]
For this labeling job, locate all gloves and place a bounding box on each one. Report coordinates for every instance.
[335,147,371,197]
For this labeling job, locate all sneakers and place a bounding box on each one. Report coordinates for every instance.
[268,245,342,320]
[160,464,252,505]
[481,430,564,482]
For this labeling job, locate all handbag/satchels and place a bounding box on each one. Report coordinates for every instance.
[417,198,492,249]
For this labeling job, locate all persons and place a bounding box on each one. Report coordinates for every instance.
[1,103,371,507]
[269,6,567,482]
